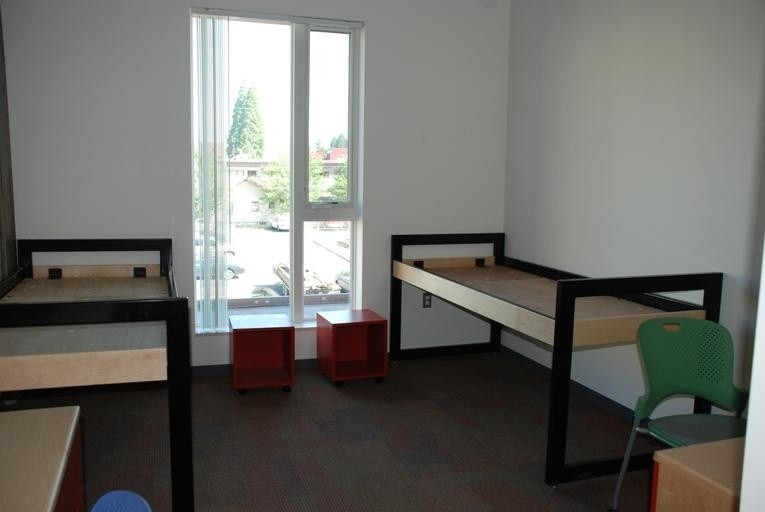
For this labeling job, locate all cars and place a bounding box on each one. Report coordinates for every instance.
[270,214,288,231]
[193,218,245,279]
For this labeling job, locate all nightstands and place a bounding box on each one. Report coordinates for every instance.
[317,309,387,388]
[229,314,296,395]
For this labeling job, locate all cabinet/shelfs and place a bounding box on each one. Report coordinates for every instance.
[0,405,86,512]
[649,437,744,512]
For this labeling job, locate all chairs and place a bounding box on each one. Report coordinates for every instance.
[273,262,342,296]
[612,316,749,510]
[336,276,351,291]
[90,489,151,512]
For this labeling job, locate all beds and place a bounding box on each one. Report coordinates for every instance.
[0,239,193,512]
[391,233,722,486]
